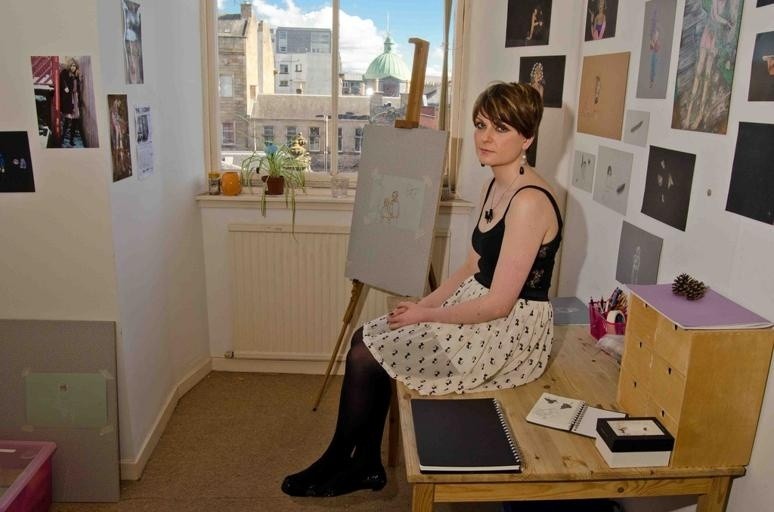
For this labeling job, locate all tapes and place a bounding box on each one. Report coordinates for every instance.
[607,310,625,335]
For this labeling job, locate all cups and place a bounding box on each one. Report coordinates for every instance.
[331,177,349,198]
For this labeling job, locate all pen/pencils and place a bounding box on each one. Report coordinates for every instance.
[606,287,628,313]
[590,296,605,320]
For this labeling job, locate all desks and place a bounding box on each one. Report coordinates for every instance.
[387,324,746,512]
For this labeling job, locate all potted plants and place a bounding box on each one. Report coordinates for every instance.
[241,140,307,244]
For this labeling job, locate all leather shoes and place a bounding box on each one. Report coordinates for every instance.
[307,466,388,497]
[281,462,329,498]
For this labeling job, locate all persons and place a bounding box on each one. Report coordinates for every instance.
[587,1,607,39]
[280,81,563,498]
[58,57,86,148]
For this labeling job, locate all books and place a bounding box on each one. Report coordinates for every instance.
[525,391,628,440]
[621,282,774,332]
[410,398,522,475]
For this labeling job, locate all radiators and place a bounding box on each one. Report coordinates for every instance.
[228,222,450,361]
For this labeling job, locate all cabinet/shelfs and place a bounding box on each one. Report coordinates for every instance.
[616,292,774,468]
